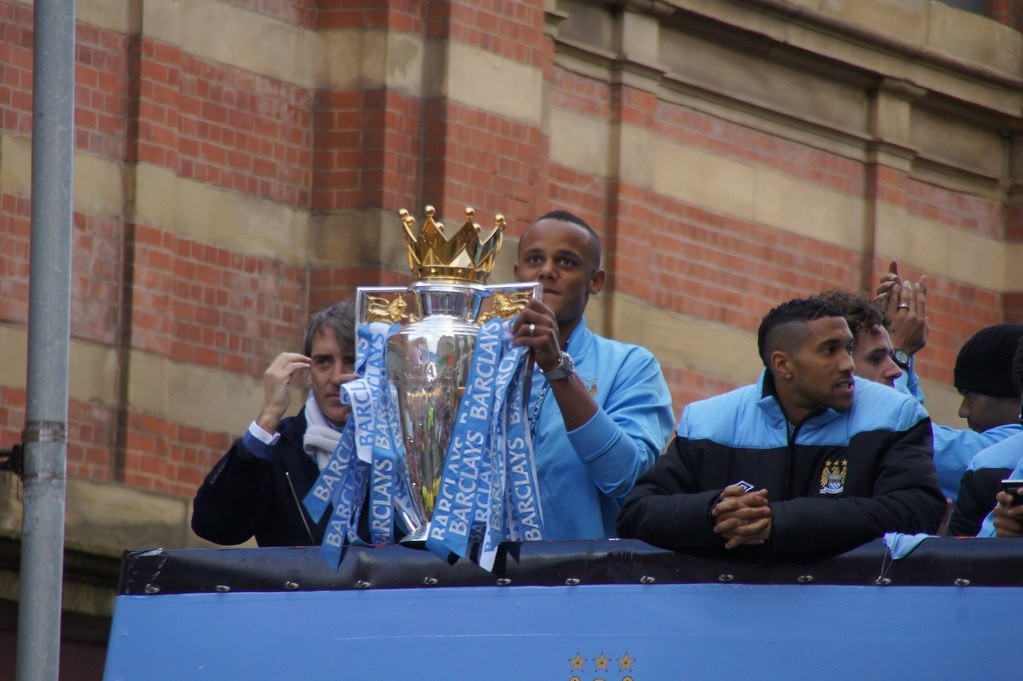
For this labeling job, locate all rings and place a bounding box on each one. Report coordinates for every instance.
[898,303,909,308]
[530,324,535,336]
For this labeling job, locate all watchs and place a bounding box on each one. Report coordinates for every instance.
[893,347,912,369]
[545,351,575,381]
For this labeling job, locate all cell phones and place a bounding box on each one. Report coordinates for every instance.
[1002,480,1023,507]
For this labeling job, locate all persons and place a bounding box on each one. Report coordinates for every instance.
[613,262,1023,548]
[511,209,676,539]
[192,300,369,548]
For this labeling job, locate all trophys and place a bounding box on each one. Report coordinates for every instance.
[302,205,551,571]
